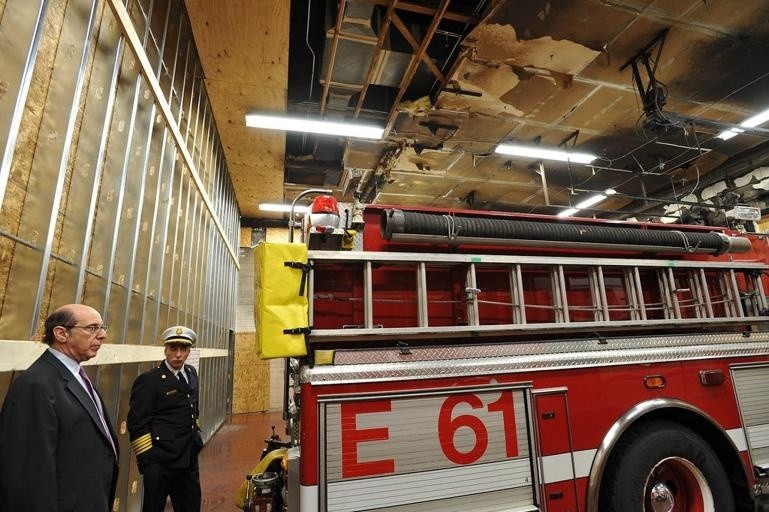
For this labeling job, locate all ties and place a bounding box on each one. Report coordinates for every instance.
[177,372,188,390]
[79,367,119,463]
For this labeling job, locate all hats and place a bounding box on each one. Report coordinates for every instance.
[161,326,197,345]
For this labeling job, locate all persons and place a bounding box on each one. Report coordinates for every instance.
[126,325,205,512]
[1,302,122,512]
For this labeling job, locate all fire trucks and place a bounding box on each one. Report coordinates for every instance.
[244,183,769,512]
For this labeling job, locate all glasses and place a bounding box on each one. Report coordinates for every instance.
[63,325,108,332]
